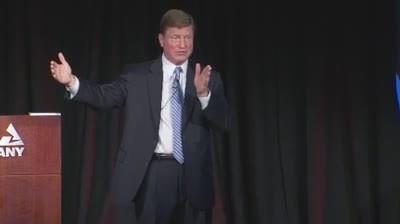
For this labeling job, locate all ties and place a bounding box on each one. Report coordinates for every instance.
[171,67,184,163]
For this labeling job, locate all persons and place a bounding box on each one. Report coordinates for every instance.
[51,9,229,224]
